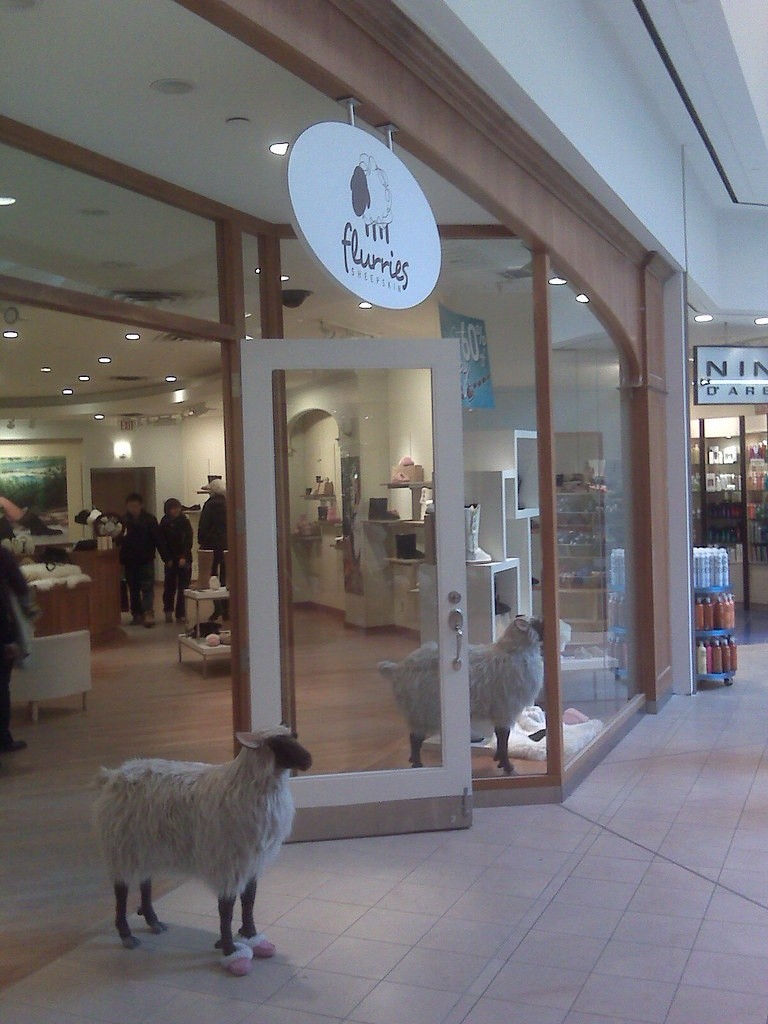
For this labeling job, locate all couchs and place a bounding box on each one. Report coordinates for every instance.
[9,629,93,721]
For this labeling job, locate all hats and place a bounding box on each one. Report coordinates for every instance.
[210,479,225,495]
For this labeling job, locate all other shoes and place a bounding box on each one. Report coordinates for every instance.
[177,616,187,624]
[0,741,27,750]
[208,609,229,621]
[164,612,173,622]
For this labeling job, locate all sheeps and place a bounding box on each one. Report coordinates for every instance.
[377,614,570,775]
[92,725,310,974]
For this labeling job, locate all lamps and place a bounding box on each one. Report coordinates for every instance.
[114,437,132,460]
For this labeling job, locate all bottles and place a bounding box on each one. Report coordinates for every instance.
[608,548,632,672]
[691,546,739,675]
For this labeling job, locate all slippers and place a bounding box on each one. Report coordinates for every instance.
[398,456,415,465]
[218,634,231,645]
[206,634,220,647]
[392,472,411,482]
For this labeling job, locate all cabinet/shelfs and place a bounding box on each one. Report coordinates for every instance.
[409,426,542,757]
[692,463,767,607]
[20,546,126,646]
[178,586,232,681]
[293,495,343,550]
[182,490,229,588]
[360,484,433,567]
[694,584,737,688]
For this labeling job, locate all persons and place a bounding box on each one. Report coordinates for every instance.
[197,480,229,622]
[116,493,159,628]
[156,498,194,624]
[1,515,42,752]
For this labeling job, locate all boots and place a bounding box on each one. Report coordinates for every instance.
[190,549,214,589]
[396,534,425,559]
[495,595,511,614]
[130,612,142,624]
[464,503,492,565]
[368,498,401,520]
[518,475,525,510]
[223,550,228,590]
[142,611,156,627]
